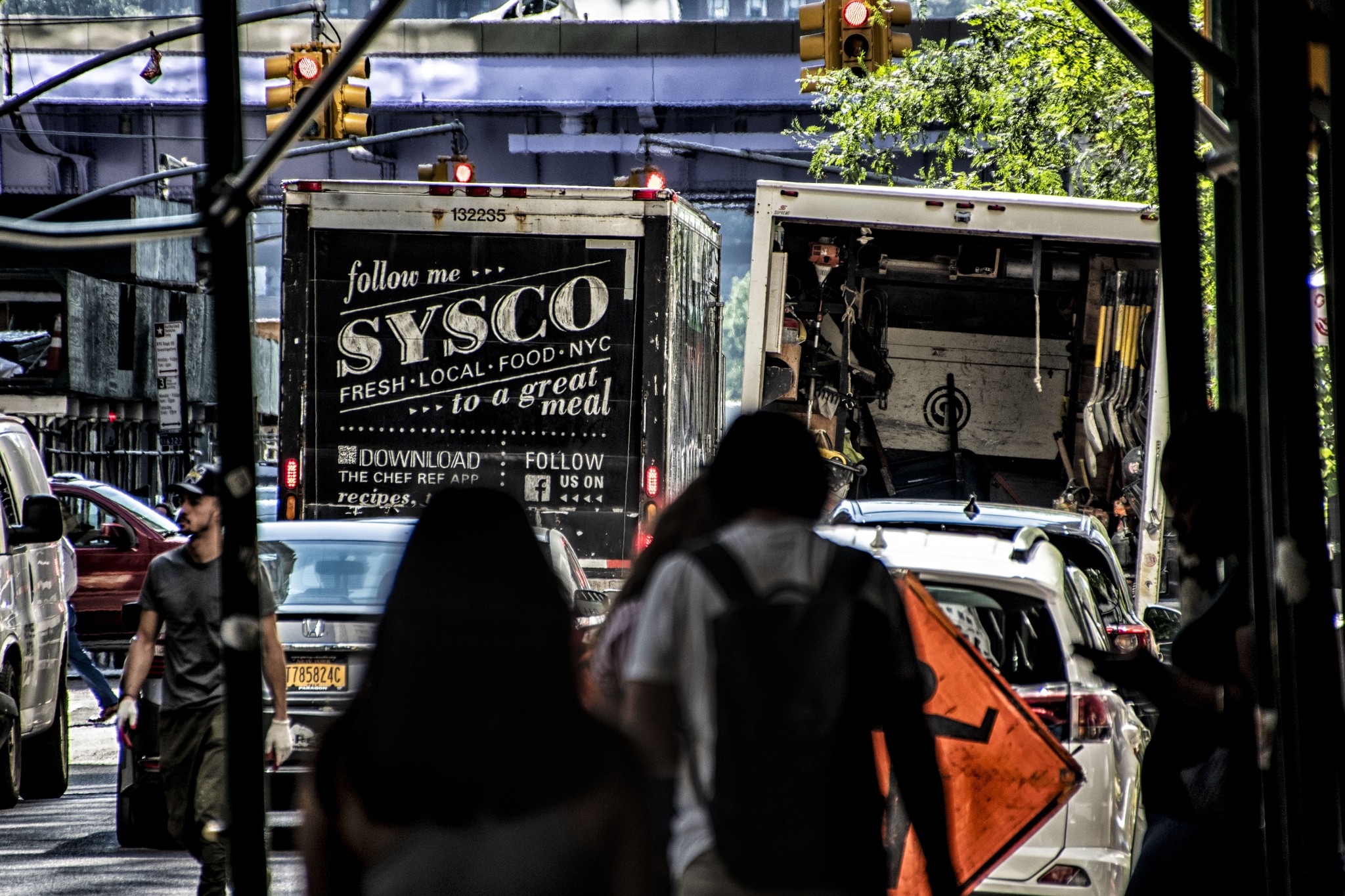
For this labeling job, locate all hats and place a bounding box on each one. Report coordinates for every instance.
[164,463,220,496]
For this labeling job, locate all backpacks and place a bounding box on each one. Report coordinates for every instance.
[674,537,890,896]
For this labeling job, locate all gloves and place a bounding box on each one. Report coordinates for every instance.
[115,699,137,749]
[263,719,293,770]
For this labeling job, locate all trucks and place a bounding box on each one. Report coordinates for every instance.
[274,176,726,613]
[738,174,1173,632]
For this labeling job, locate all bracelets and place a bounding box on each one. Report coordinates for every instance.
[119,694,136,701]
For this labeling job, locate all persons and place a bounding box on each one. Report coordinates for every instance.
[1093,409,1250,896]
[117,462,293,896]
[300,408,965,896]
[61,535,119,722]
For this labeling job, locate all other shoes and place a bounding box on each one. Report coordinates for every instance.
[88,701,118,723]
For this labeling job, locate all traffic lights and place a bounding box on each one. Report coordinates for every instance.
[646,172,664,190]
[416,162,446,182]
[798,0,843,94]
[289,51,329,141]
[840,0,876,83]
[331,51,373,140]
[263,53,292,140]
[612,174,641,187]
[878,0,913,73]
[447,162,477,183]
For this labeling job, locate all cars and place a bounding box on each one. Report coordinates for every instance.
[0,412,87,782]
[46,470,192,634]
[113,515,612,853]
[814,496,1164,896]
